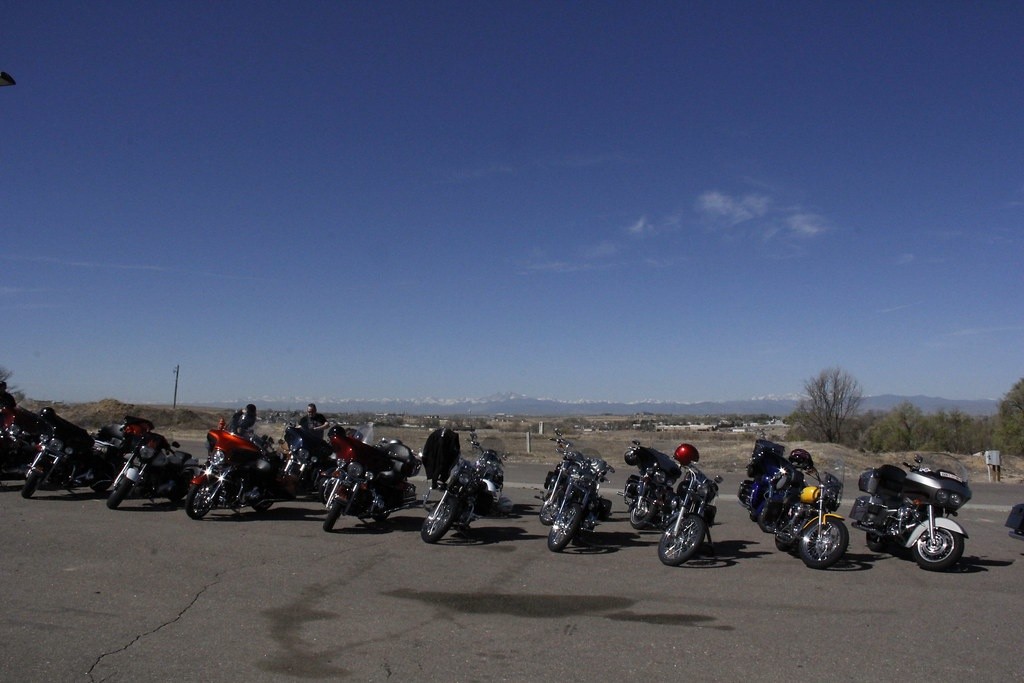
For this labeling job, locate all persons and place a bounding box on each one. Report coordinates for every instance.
[299,403,329,439]
[230,404,256,435]
[0,381,16,437]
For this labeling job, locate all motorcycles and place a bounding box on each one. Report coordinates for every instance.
[420,456,485,543]
[0,400,125,500]
[848,452,972,572]
[465,432,511,516]
[319,423,423,532]
[737,439,849,570]
[644,461,724,567]
[103,415,200,510]
[1007,501,1024,542]
[616,438,684,530]
[278,418,364,497]
[185,419,285,520]
[533,428,615,552]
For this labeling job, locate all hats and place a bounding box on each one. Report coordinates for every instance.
[0,381,6,389]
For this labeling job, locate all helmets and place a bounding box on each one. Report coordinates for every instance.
[591,458,607,476]
[240,414,256,428]
[789,449,813,469]
[497,496,513,514]
[39,407,56,422]
[625,450,641,466]
[674,443,699,466]
[328,426,345,439]
